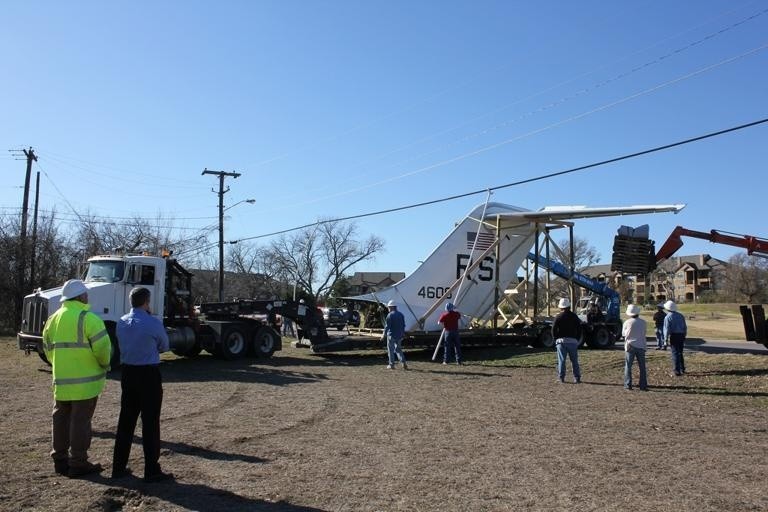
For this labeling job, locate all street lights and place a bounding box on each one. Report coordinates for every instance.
[219,199,256,302]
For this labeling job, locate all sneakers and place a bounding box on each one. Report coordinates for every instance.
[144,470,173,482]
[54,459,69,473]
[111,467,132,478]
[441,358,464,365]
[69,458,102,475]
[386,364,395,370]
[402,363,408,370]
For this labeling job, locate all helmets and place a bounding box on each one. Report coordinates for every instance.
[557,298,571,309]
[656,303,664,309]
[387,299,397,307]
[60,280,91,301]
[444,302,454,311]
[664,300,677,310]
[626,303,641,316]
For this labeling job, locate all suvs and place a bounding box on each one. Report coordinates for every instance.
[316,306,347,330]
[342,310,360,328]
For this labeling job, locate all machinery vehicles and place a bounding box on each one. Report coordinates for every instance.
[612,225,767,350]
[526,252,622,349]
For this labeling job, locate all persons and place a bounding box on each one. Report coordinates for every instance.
[116,287,173,483]
[551,298,581,384]
[653,303,667,350]
[622,304,647,390]
[385,300,407,369]
[663,300,686,376]
[440,303,461,366]
[43,280,114,477]
[587,303,604,325]
[266,311,296,338]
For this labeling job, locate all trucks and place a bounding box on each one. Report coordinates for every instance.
[16,248,623,372]
